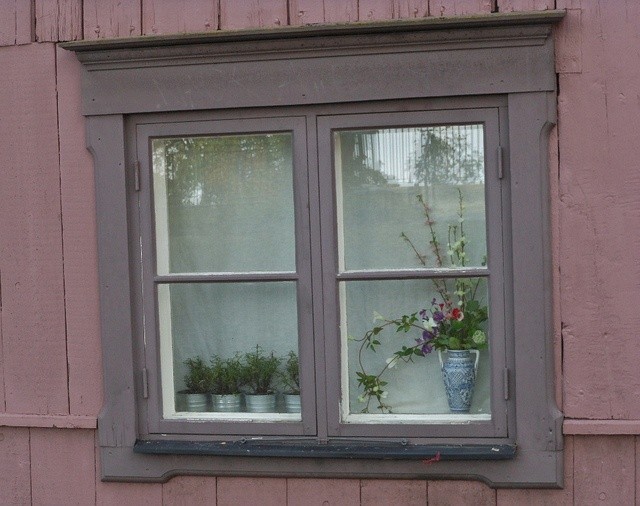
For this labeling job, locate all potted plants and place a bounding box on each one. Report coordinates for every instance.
[276,351,302,412]
[243,344,279,412]
[208,352,245,413]
[177,355,213,412]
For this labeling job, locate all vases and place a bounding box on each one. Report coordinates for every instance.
[438,348,480,411]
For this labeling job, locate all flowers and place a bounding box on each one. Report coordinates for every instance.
[348,188,488,402]
